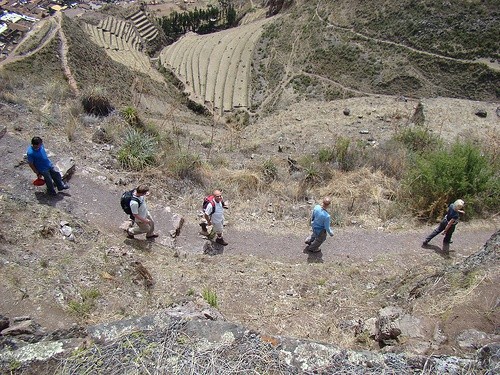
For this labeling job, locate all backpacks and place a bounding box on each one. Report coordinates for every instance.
[202,194,225,214]
[120,189,141,214]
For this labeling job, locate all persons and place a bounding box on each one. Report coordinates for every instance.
[26,136,69,196]
[422,199,465,247]
[205,188,229,246]
[304,197,334,252]
[126,185,159,240]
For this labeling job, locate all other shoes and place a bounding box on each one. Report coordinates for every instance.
[146,233,158,238]
[216,237,228,245]
[63,184,70,189]
[443,239,453,243]
[126,227,135,238]
[199,222,207,231]
[304,239,321,252]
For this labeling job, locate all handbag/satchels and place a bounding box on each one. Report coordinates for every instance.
[34,175,46,185]
